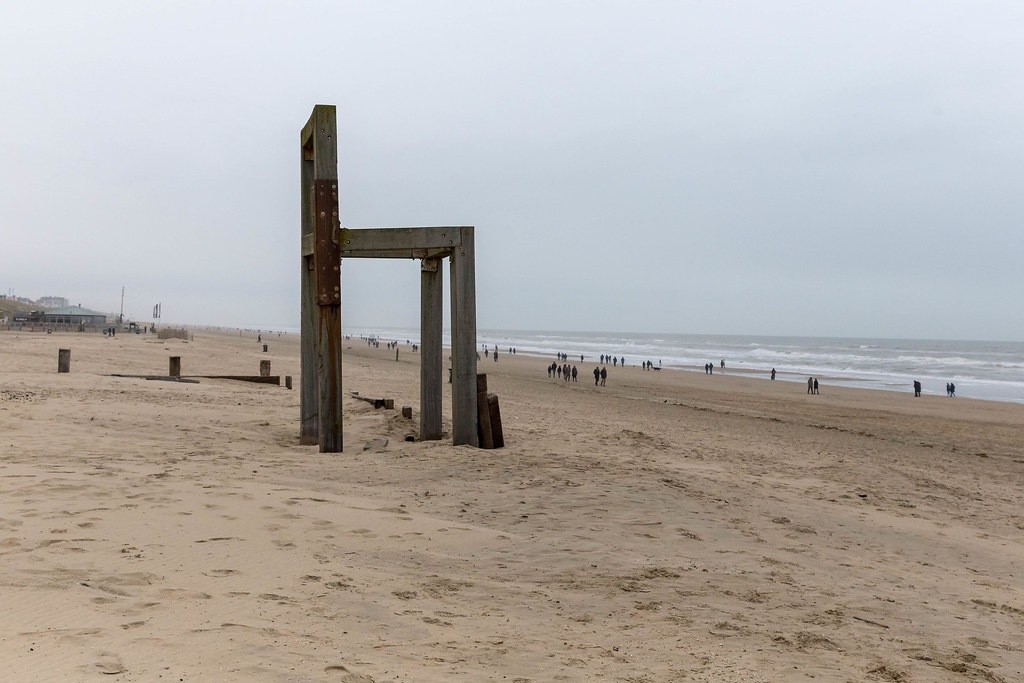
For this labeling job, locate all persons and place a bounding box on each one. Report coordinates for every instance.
[806,376,814,395]
[360,334,780,390]
[950,383,955,396]
[946,382,951,396]
[80,320,357,354]
[913,380,920,397]
[813,378,820,395]
